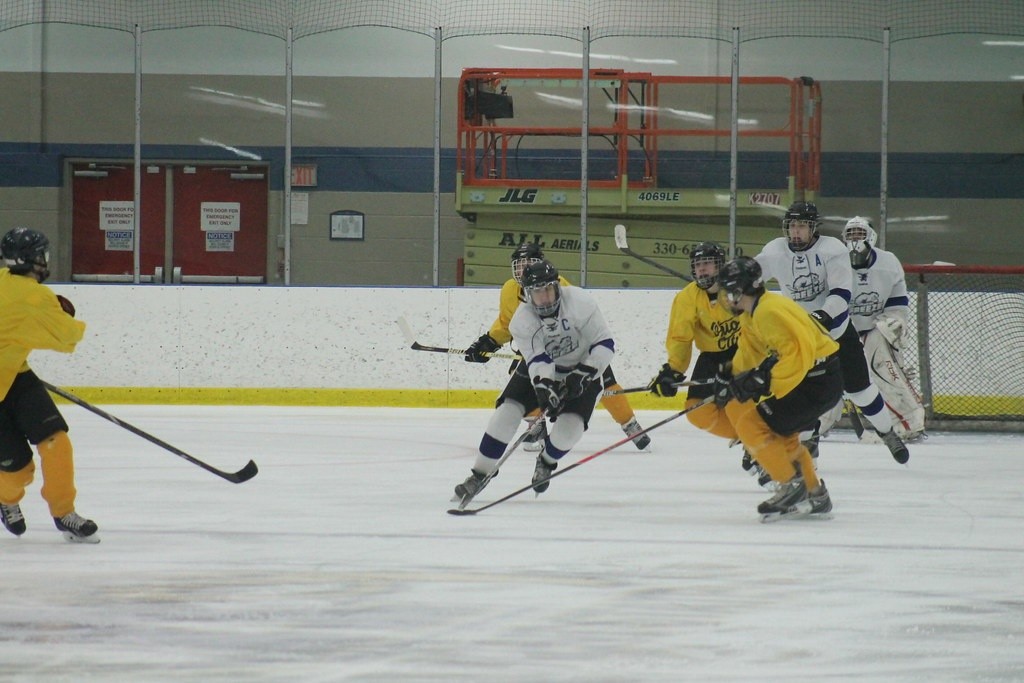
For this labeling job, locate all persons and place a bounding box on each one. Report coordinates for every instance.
[451,261,615,503]
[645,241,778,493]
[463,242,651,452]
[0,227,101,544]
[716,257,845,521]
[754,199,909,467]
[842,216,926,443]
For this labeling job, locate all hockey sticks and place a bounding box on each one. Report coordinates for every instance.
[459,395,715,515]
[601,378,716,397]
[39,378,259,484]
[396,315,523,360]
[841,393,884,444]
[458,388,569,512]
[614,225,695,282]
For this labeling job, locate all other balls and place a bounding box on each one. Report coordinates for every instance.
[447,509,462,515]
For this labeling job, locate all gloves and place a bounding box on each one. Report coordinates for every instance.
[648,363,686,396]
[811,310,832,331]
[714,371,735,408]
[546,373,584,422]
[731,367,771,402]
[465,331,501,362]
[532,374,560,415]
[58,295,75,316]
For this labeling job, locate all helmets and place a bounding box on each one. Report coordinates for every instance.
[783,201,819,252]
[690,241,725,289]
[521,260,558,288]
[842,217,877,267]
[511,244,544,284]
[0,227,49,283]
[718,256,763,295]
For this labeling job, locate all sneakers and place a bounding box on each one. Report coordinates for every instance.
[803,421,821,471]
[758,469,779,491]
[758,461,806,523]
[450,467,499,504]
[54,510,100,544]
[742,445,758,476]
[522,418,548,451]
[622,416,651,452]
[780,479,833,520]
[875,427,909,464]
[0,503,26,536]
[532,449,558,498]
